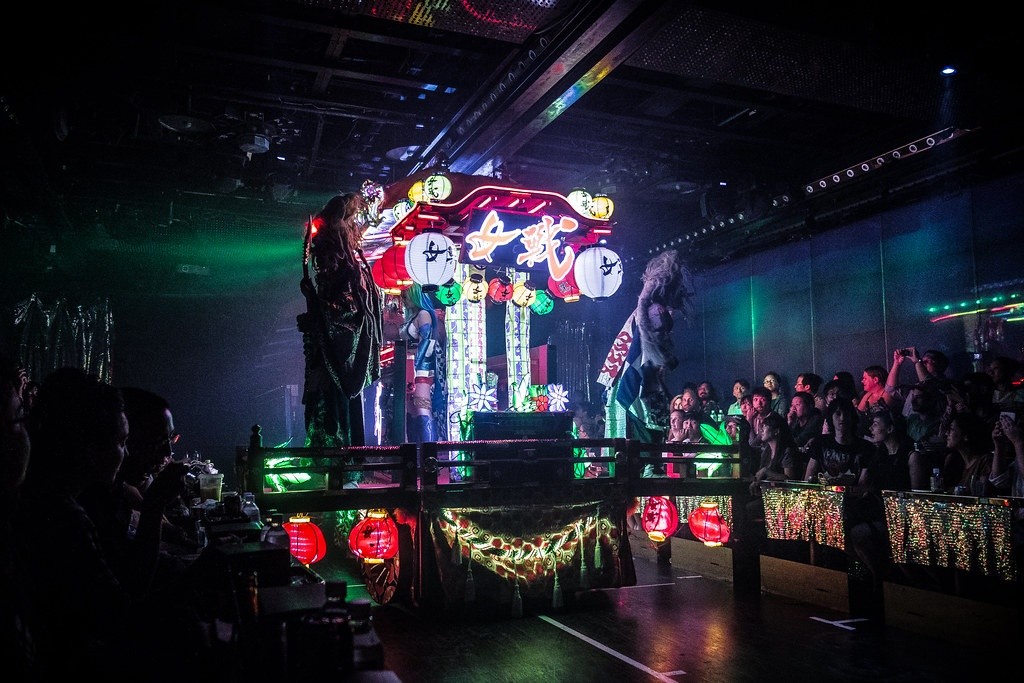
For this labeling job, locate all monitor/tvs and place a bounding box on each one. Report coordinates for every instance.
[471,412,576,482]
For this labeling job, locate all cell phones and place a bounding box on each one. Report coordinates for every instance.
[1000,412,1016,422]
[972,353,985,359]
[900,350,911,357]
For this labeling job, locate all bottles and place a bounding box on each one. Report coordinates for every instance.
[320,580,352,630]
[261,509,291,585]
[930,468,943,491]
[336,599,386,678]
[240,492,261,523]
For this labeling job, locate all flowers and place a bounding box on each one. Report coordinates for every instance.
[536,384,572,412]
[468,384,499,412]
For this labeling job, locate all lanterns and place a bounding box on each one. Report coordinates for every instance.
[687,498,731,547]
[372,174,623,316]
[346,509,399,565]
[282,512,326,568]
[642,495,679,541]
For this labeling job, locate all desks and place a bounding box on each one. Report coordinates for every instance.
[174,500,402,683]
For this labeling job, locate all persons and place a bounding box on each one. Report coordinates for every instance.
[0,365,206,683]
[570,389,607,477]
[663,345,1024,607]
[287,191,382,488]
[615,249,694,478]
[398,281,437,477]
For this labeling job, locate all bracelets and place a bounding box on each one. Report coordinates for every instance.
[913,358,922,367]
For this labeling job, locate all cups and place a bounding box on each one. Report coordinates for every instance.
[198,473,224,503]
